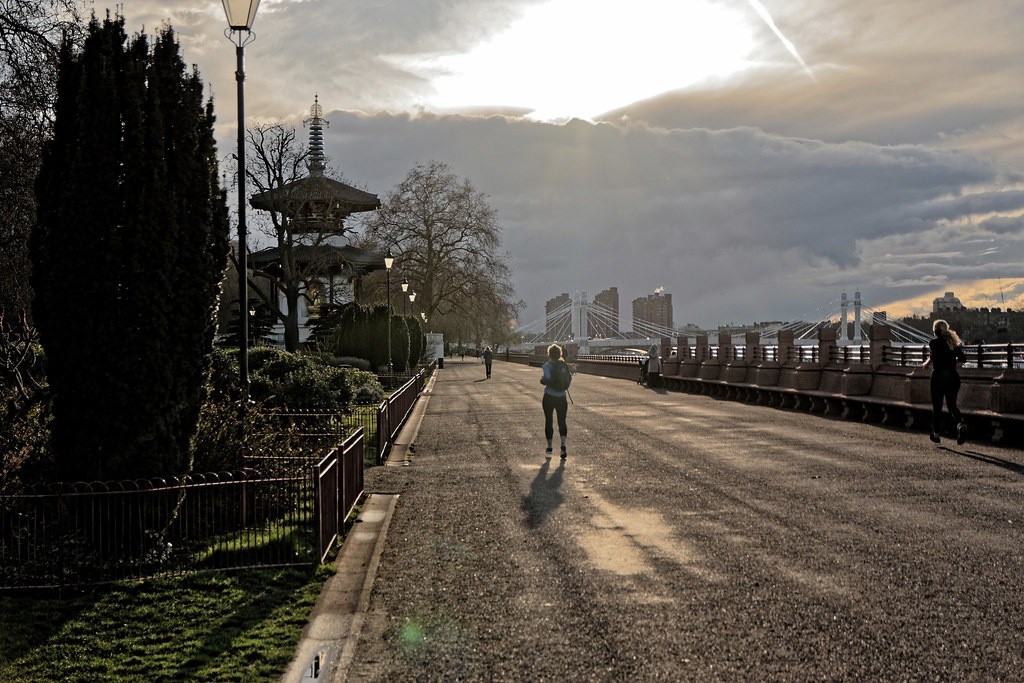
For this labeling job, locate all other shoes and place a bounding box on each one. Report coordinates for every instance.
[487,376,491,379]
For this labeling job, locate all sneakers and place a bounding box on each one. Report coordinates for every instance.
[560,443,567,459]
[957,424,966,445]
[930,433,941,445]
[546,447,553,454]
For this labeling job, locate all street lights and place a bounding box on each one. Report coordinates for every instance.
[407,289,417,315]
[383,248,395,392]
[420,309,428,337]
[401,275,409,318]
[221,0,261,530]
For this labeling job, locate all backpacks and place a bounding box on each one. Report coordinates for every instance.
[549,360,573,404]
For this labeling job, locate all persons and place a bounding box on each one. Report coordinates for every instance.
[447,347,456,359]
[506,348,509,361]
[461,349,464,361]
[540,344,572,457]
[923,321,967,445]
[647,344,659,388]
[476,347,479,360]
[480,346,494,379]
